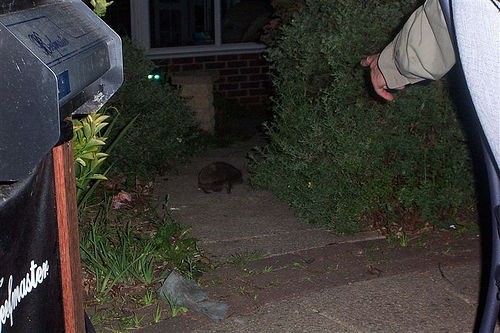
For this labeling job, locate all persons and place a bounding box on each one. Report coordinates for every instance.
[356,0,500,103]
